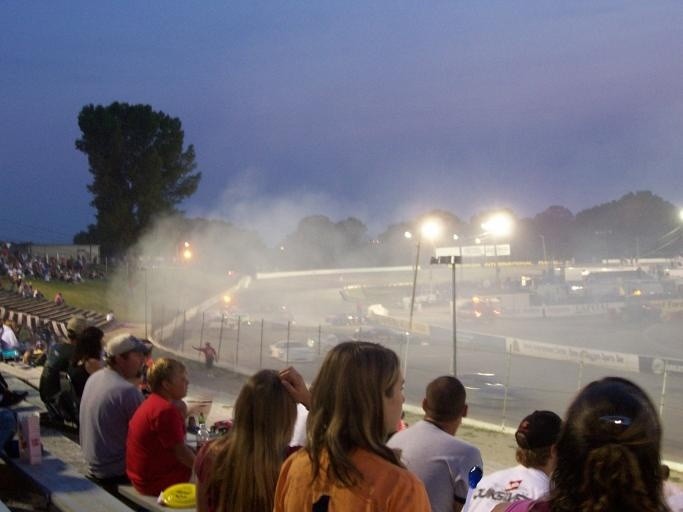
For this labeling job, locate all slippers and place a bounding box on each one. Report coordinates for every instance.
[44,372,80,434]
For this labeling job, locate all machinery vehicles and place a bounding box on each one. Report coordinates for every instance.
[265,337,321,365]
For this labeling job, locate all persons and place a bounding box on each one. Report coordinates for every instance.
[192,342,217,367]
[3,314,199,495]
[489,377,674,511]
[273,339,433,512]
[191,364,313,512]
[386,373,483,511]
[465,411,564,510]
[3,241,105,306]
[661,463,678,501]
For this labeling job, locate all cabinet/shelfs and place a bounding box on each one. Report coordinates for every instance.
[189,411,209,447]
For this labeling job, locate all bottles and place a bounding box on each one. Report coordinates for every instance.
[515,410,561,448]
[67,318,96,337]
[103,333,154,362]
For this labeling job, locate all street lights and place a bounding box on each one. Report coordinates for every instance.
[18,412,40,465]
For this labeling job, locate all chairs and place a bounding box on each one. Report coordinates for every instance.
[0,391,28,407]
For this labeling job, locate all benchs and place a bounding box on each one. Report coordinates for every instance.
[1,286,210,512]
